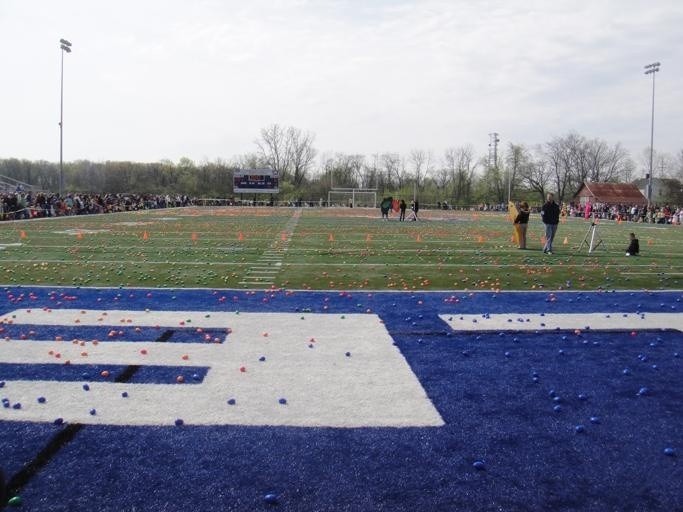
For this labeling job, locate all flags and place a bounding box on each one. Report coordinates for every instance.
[390,200,400,213]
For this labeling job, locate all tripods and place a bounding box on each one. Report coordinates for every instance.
[405,211,419,221]
[578,226,609,257]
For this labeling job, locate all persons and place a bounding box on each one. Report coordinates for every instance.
[411,199,418,222]
[378,196,392,221]
[434,199,682,226]
[511,201,529,249]
[625,232,639,256]
[539,191,561,256]
[398,199,406,221]
[348,196,352,208]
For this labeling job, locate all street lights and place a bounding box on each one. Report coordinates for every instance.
[57,36,74,198]
[642,59,660,211]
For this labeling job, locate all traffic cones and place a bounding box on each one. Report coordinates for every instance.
[475,235,484,242]
[425,211,624,227]
[327,233,335,241]
[415,233,422,242]
[19,229,27,238]
[646,239,654,248]
[237,232,245,241]
[562,237,569,244]
[280,231,287,240]
[190,230,197,241]
[76,232,84,240]
[364,232,372,240]
[141,230,149,239]
